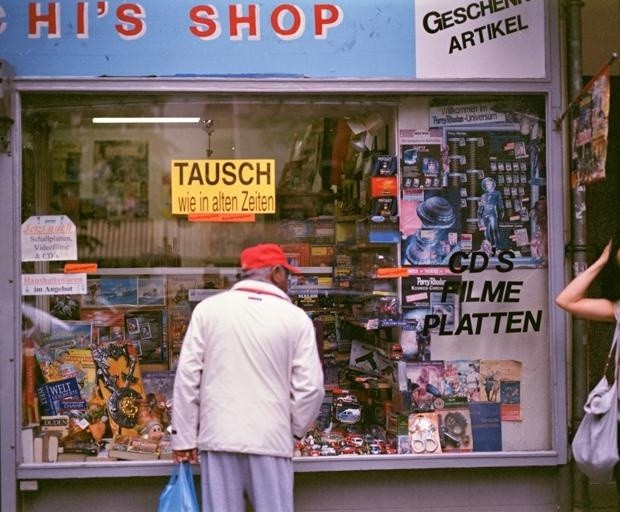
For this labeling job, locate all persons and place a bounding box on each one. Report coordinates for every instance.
[478,175,506,253]
[426,158,441,174]
[557,232,619,498]
[168,243,327,510]
[530,196,548,266]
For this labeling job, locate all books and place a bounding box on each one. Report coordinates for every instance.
[21,339,90,464]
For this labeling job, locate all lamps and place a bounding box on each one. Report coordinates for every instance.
[83,115,203,127]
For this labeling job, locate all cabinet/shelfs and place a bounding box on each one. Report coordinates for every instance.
[347,243,402,331]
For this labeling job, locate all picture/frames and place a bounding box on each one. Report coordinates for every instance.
[80,278,167,363]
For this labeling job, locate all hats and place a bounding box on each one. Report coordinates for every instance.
[240,244,300,273]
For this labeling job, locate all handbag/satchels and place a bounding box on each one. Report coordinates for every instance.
[572,376,619,483]
